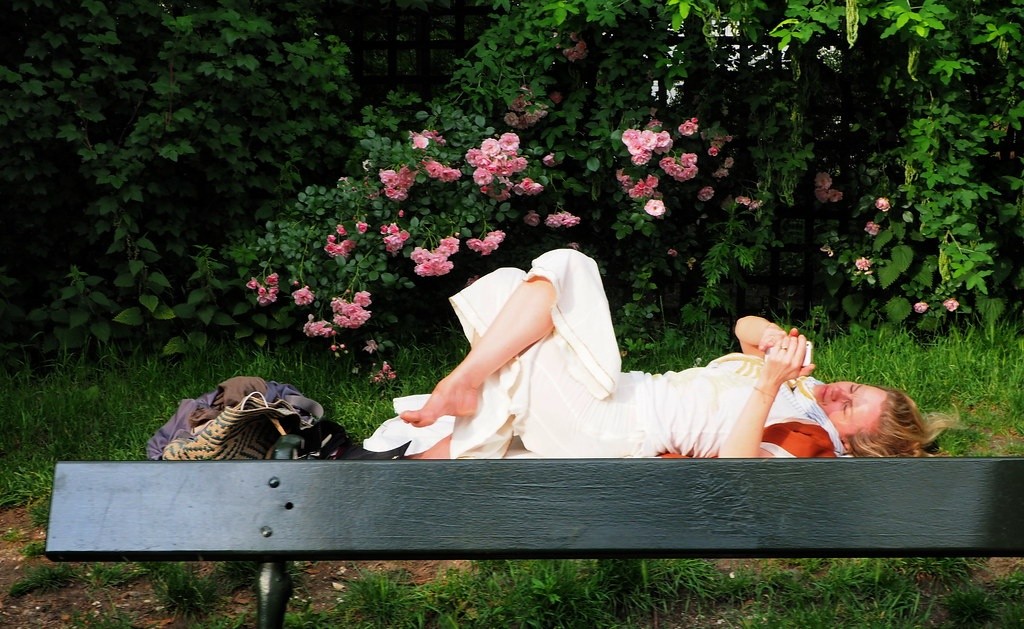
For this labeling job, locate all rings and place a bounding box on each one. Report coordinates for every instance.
[780,346,787,350]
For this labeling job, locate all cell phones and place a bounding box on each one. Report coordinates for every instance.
[765,341,814,367]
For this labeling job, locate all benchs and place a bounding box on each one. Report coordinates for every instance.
[45,458,1024,629]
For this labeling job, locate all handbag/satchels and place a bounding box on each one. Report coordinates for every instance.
[162,392,312,461]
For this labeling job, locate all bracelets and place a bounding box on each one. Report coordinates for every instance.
[753,385,777,400]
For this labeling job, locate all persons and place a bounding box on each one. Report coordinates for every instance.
[363,247,957,458]
